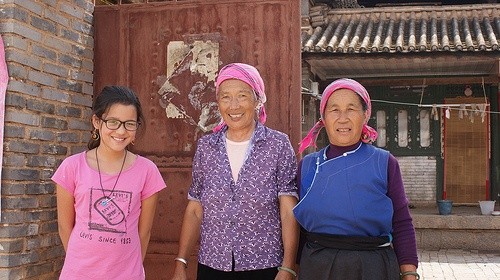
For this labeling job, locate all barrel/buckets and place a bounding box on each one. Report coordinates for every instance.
[479,200,496,215]
[437,199,453,215]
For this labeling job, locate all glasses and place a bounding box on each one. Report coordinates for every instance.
[96,115,141,131]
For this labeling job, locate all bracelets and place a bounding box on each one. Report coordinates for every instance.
[278,267,297,278]
[399,270,420,280]
[174,257,188,268]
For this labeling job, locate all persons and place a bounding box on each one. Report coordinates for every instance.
[296,78,419,280]
[51,86,167,280]
[171,63,299,280]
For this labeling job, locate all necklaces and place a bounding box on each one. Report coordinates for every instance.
[96,146,128,207]
